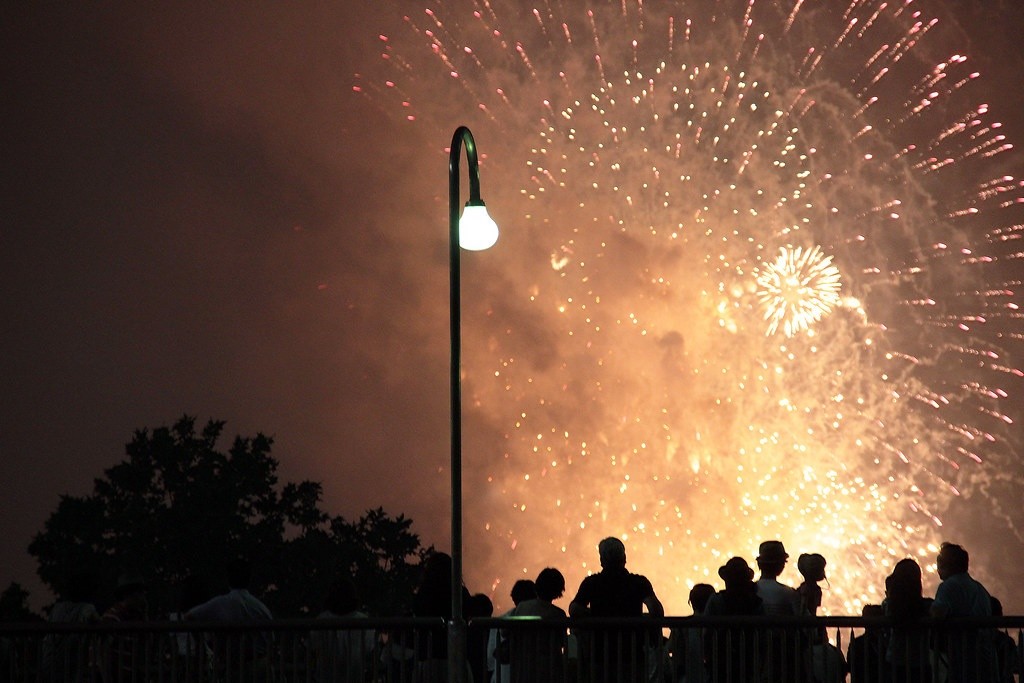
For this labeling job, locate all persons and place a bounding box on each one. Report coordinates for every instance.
[847,543,1024,683]
[180,569,278,682]
[308,568,494,683]
[39,595,147,683]
[669,541,847,683]
[567,538,669,683]
[491,569,569,683]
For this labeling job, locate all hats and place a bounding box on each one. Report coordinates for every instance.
[798,553,825,581]
[755,541,789,564]
[718,557,754,582]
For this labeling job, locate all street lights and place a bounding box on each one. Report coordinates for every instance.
[448,126,501,582]
